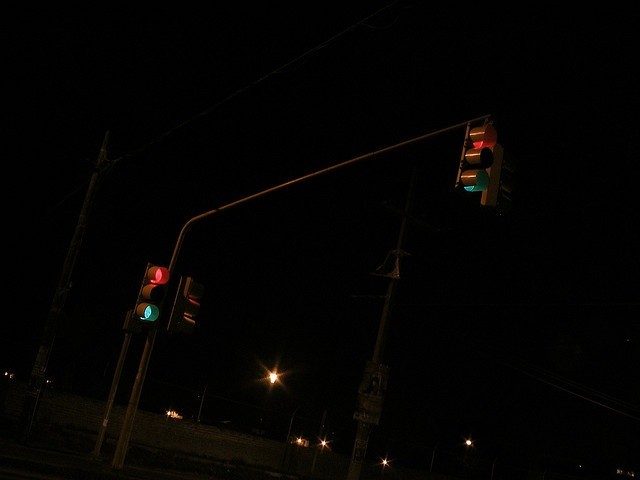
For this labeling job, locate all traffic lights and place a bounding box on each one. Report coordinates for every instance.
[460,122,498,191]
[176,275,205,335]
[133,264,173,323]
